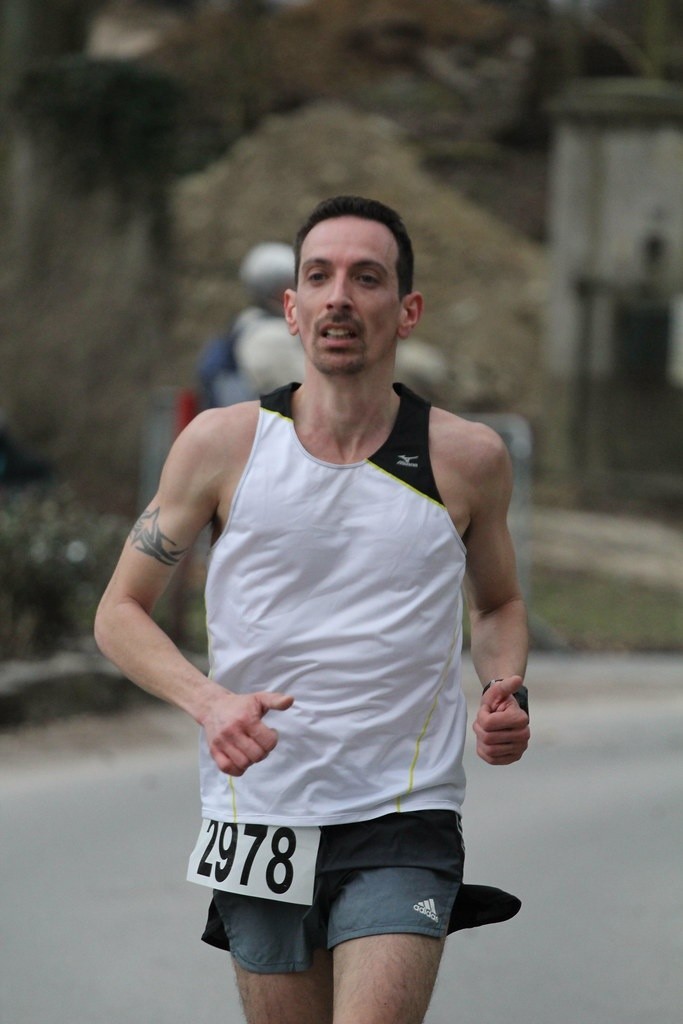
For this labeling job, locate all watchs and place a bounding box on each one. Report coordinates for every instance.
[482,679,529,715]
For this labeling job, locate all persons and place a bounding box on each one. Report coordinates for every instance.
[609,242,670,388]
[197,241,306,404]
[95,196,531,1024]
